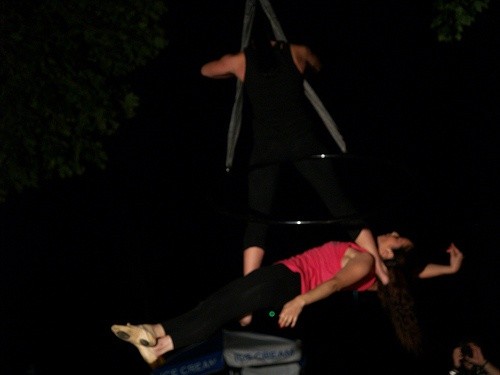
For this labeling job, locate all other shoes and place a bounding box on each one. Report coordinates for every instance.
[113,325,156,346]
[136,344,156,364]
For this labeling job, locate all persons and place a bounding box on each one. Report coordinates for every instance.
[448,342,500,375]
[200,15,390,327]
[112,231,464,365]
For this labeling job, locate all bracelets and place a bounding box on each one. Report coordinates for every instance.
[482,359,488,367]
